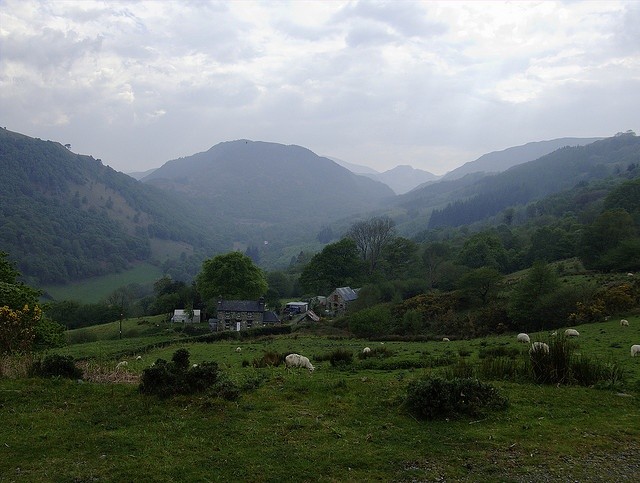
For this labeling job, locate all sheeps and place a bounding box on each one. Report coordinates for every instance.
[631,344,640,357]
[285,354,315,375]
[517,333,531,343]
[564,329,580,339]
[528,342,550,354]
[620,319,628,326]
